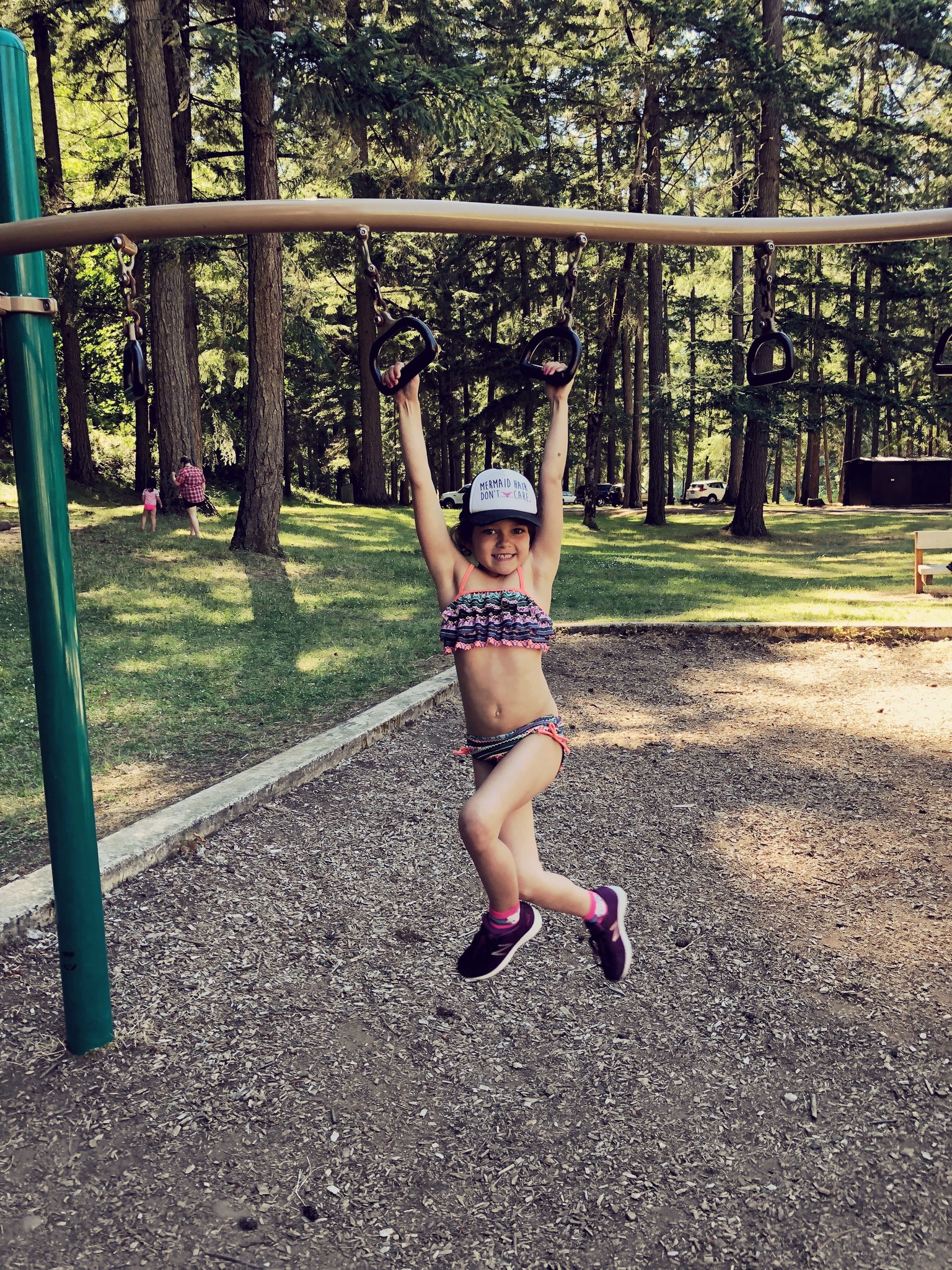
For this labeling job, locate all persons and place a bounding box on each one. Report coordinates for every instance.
[172,456,205,538]
[141,477,163,532]
[378,361,634,984]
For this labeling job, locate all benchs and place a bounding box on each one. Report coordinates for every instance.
[914,531,952,594]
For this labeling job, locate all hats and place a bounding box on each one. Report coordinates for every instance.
[459,468,542,528]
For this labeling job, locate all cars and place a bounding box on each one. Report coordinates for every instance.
[562,491,579,505]
[439,482,472,510]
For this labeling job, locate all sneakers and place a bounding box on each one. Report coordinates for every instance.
[456,901,542,980]
[586,885,631,982]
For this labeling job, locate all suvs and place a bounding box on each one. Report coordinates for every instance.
[685,479,728,507]
[575,482,615,507]
[607,482,648,507]
